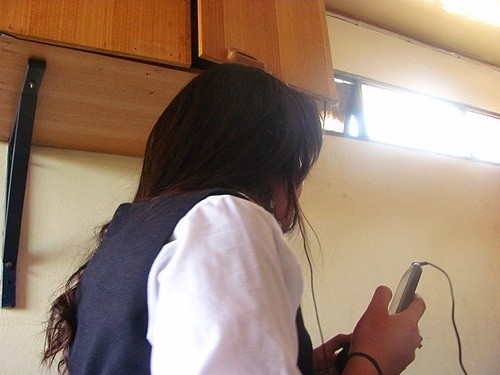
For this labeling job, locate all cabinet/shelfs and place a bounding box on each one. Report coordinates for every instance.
[1,0,340,168]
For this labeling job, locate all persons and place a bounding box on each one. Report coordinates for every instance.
[60,64,426,375]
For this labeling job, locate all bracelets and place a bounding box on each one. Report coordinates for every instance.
[345,350,387,374]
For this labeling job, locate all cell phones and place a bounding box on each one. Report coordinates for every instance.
[389,262,423,316]
